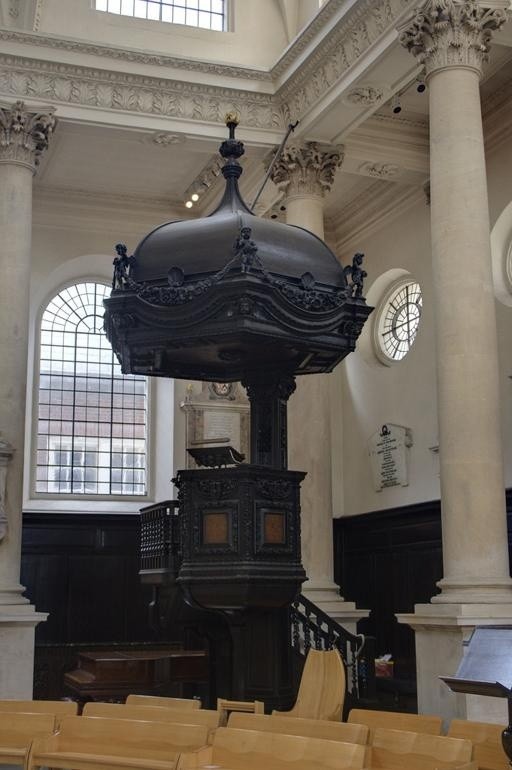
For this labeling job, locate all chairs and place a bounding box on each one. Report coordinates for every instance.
[271,649,346,722]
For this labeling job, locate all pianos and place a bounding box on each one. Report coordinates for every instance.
[62,646,212,709]
